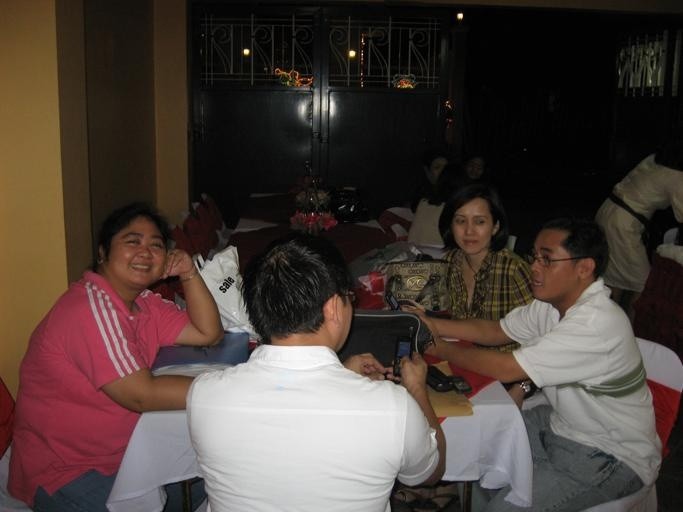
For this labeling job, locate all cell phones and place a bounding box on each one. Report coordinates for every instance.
[385,290,402,311]
[450,376,472,393]
[393,337,413,376]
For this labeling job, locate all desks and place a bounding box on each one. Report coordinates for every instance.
[105,193,535,511]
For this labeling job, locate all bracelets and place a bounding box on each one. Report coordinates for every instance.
[179,271,197,282]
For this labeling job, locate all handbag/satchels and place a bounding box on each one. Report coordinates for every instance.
[337,310,433,380]
[385,254,452,319]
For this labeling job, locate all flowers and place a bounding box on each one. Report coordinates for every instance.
[289,179,337,236]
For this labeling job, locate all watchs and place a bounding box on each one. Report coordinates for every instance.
[518,380,531,394]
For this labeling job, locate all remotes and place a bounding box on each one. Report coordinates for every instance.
[426,366,453,392]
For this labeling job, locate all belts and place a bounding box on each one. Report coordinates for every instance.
[608,193,649,227]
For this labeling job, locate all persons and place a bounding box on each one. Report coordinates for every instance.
[411,183,538,410]
[5,200,224,512]
[593,145,682,336]
[183,231,446,512]
[405,148,486,248]
[398,215,666,512]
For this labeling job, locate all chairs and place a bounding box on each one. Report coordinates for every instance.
[0,377,31,512]
[154,193,234,307]
[583,337,683,511]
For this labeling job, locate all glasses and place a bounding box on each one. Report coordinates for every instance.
[527,253,591,266]
